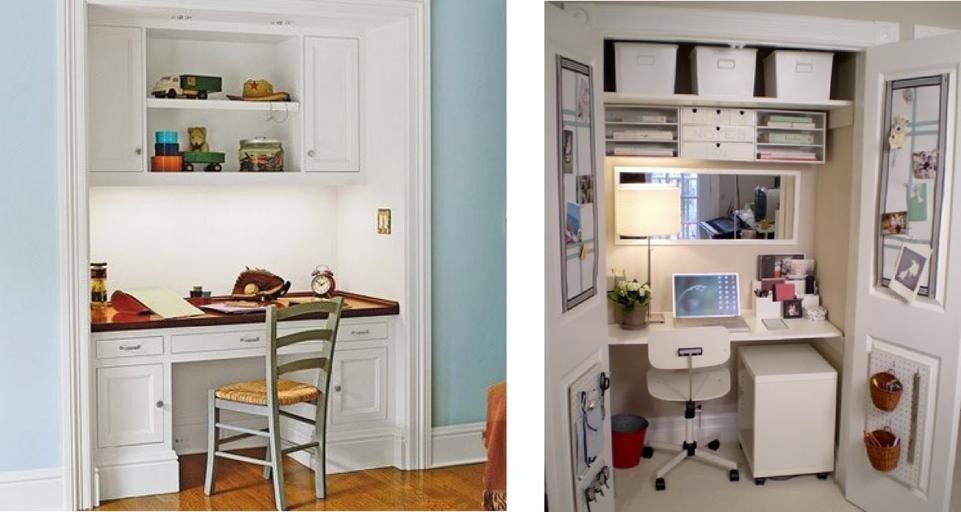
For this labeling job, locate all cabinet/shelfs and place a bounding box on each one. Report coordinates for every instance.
[91,315,400,501]
[86,12,365,186]
[605,104,827,164]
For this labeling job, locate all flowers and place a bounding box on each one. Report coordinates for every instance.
[604,268,654,312]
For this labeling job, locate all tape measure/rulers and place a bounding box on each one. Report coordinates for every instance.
[905,373,920,465]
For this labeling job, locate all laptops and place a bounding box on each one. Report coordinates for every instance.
[672,272,751,332]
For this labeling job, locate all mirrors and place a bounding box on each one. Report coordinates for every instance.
[615,166,803,244]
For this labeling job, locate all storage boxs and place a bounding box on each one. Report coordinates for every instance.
[764,51,833,98]
[612,40,678,95]
[689,46,758,97]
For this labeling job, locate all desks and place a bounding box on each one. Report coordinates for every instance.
[734,210,774,239]
[734,344,838,487]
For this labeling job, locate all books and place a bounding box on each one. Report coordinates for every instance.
[759,115,818,164]
[200,296,352,315]
[614,115,675,158]
[758,253,817,304]
[111,284,207,318]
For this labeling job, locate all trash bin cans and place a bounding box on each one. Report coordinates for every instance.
[607,413,648,469]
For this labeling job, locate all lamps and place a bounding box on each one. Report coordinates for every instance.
[615,183,681,322]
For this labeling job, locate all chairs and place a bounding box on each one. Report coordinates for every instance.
[643,326,740,489]
[205,296,345,510]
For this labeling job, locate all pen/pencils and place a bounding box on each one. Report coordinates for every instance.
[863,429,900,448]
[754,288,769,298]
[873,378,902,392]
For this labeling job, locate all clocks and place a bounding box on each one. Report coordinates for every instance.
[311,264,335,298]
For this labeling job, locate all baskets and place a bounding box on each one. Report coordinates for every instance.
[865,427,899,471]
[870,369,903,412]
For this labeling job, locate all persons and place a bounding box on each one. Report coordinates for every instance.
[189,127,211,153]
[566,214,580,243]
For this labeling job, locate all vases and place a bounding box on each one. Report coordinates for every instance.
[622,305,645,331]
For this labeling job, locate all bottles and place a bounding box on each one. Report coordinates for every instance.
[89,263,107,310]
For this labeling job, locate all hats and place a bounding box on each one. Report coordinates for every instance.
[226,80,290,101]
